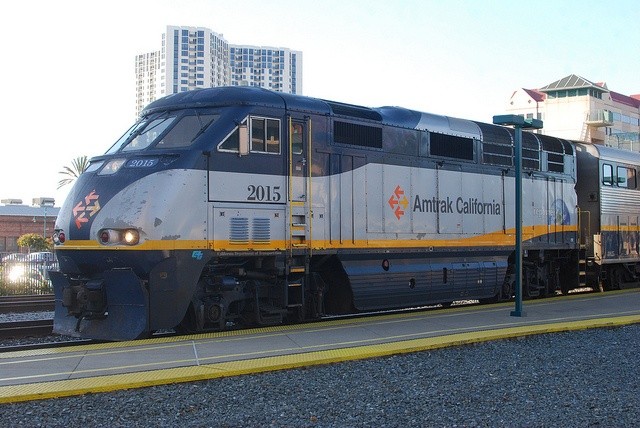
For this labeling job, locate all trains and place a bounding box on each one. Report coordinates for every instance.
[47,85,640,342]
